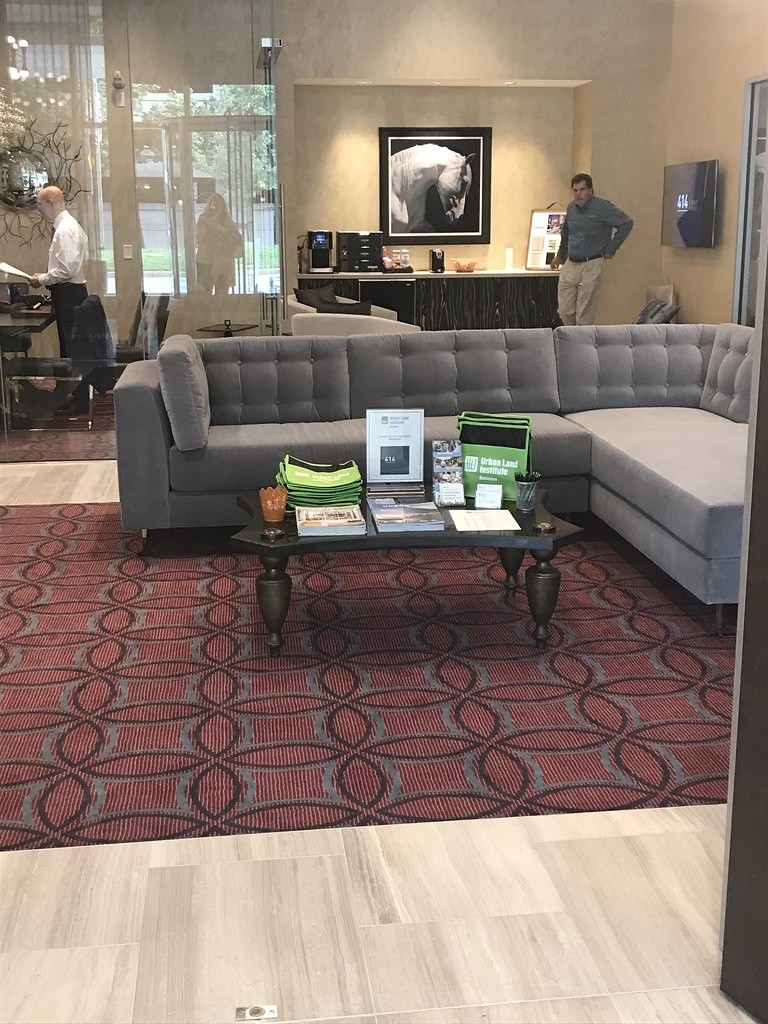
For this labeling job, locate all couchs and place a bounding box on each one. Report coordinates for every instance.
[110,321,763,633]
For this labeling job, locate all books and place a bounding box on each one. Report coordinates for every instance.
[367,498,444,532]
[296,504,367,536]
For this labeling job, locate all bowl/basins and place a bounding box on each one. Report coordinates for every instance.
[451,258,478,272]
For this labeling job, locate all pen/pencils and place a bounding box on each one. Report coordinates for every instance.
[514,471,543,510]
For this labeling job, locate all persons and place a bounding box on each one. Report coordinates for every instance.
[195,193,236,294]
[28,186,89,415]
[551,173,633,326]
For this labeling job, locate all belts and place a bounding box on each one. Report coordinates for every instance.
[569,254,603,263]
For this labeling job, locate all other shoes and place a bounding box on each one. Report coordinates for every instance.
[56,401,89,416]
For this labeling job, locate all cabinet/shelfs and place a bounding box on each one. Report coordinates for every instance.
[415,277,560,330]
[299,278,360,299]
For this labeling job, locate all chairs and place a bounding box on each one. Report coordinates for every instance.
[292,302,421,338]
[287,288,398,322]
[87,290,170,418]
[636,282,682,327]
[1,294,114,434]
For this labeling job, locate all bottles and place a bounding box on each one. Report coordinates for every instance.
[10,283,20,304]
[402,249,409,267]
[393,250,400,264]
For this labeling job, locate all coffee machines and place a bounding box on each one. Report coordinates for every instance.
[307,230,334,272]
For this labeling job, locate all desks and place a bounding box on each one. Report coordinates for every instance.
[0,300,62,430]
[197,322,258,338]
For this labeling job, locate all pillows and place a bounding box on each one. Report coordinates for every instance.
[292,283,337,307]
[155,333,213,452]
[633,300,677,326]
[314,301,372,317]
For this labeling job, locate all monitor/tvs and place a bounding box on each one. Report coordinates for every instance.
[661,158,719,248]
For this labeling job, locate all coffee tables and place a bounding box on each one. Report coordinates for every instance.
[233,480,585,655]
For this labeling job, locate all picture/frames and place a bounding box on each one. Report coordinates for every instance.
[379,127,493,244]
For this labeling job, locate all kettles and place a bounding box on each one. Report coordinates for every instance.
[297,246,302,273]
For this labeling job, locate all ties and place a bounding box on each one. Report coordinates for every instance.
[46,226,56,273]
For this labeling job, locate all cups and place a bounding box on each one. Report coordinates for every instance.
[514,480,541,511]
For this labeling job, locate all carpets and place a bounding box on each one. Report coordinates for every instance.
[0,500,742,854]
[0,376,124,463]
